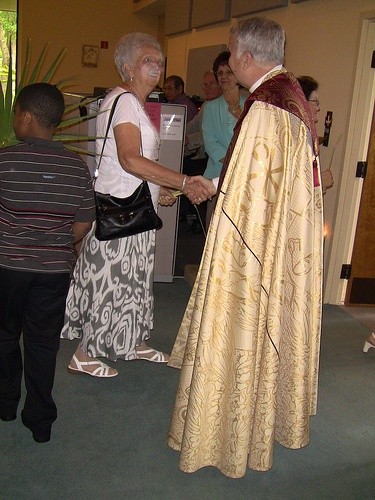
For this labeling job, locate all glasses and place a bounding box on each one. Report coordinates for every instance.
[308,99,320,106]
[132,57,163,67]
[215,70,233,76]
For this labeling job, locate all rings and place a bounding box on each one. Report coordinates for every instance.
[194,199,197,201]
[198,198,201,200]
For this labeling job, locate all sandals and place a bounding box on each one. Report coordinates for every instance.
[136,348,169,363]
[67,353,119,378]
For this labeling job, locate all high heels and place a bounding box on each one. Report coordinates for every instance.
[363,333,375,354]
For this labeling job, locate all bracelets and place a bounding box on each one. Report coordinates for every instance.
[181,174,187,191]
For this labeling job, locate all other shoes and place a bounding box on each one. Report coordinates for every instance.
[22,409,49,443]
[0,414,16,421]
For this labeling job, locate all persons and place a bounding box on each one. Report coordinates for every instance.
[296,76,333,195]
[201,50,249,234]
[163,75,198,124]
[1,82,96,443]
[168,16,323,478]
[60,33,212,378]
[184,70,222,160]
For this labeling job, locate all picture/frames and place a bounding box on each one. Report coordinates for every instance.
[82,45,99,68]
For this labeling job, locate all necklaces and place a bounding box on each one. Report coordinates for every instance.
[227,101,239,113]
[126,83,144,109]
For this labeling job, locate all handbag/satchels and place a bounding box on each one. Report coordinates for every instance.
[92,180,162,241]
